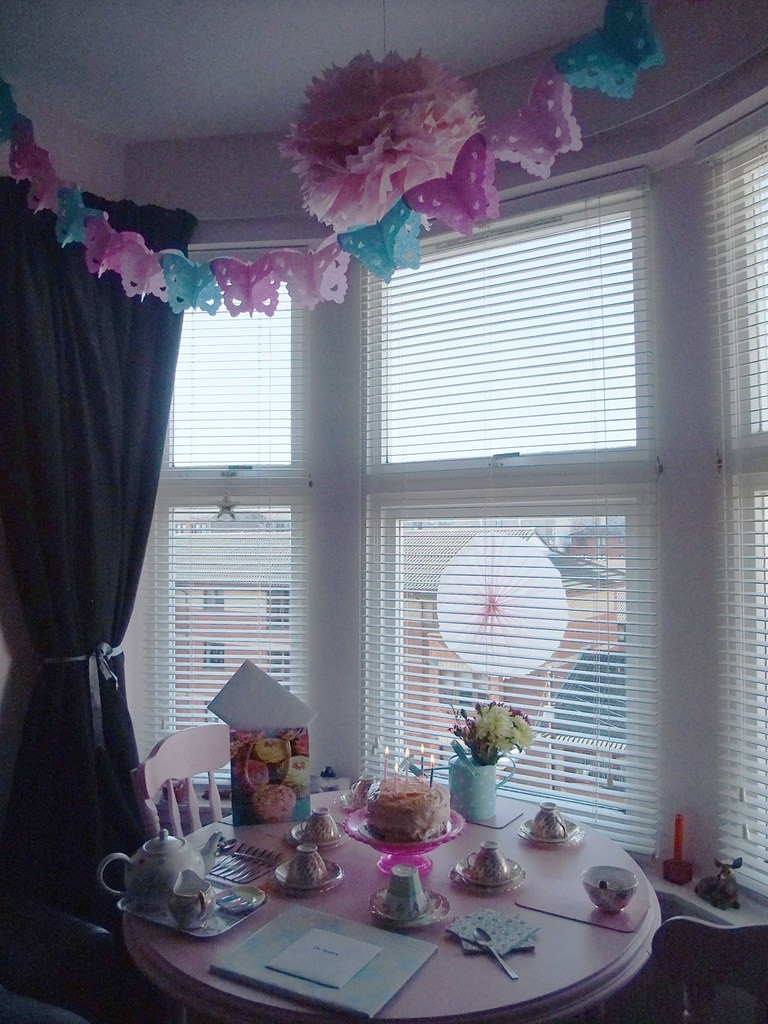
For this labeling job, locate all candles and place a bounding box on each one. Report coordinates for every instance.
[384,743,435,796]
[674,813,683,863]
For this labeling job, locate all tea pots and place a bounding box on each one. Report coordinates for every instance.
[95,828,223,913]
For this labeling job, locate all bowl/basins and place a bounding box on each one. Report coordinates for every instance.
[580,866,640,914]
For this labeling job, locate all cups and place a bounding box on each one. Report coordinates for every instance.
[530,801,569,839]
[301,807,341,847]
[384,863,429,919]
[350,774,378,806]
[469,840,511,883]
[286,844,330,887]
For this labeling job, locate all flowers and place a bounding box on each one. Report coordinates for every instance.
[278,45,488,230]
[446,701,533,766]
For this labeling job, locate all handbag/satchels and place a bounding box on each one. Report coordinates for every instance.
[231,725,312,827]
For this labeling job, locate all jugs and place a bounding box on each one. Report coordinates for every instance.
[166,868,216,928]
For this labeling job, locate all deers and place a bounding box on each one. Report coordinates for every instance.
[694,857,743,910]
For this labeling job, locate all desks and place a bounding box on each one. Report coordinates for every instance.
[122,789,662,1024]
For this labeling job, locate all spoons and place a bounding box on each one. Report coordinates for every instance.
[216,839,237,852]
[473,926,520,979]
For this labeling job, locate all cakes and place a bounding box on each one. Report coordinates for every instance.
[365,773,451,843]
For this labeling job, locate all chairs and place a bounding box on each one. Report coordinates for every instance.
[128,724,230,837]
[652,916,768,1024]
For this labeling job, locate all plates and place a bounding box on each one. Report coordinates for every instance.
[518,819,585,848]
[367,886,451,928]
[215,884,266,911]
[447,854,527,895]
[332,794,361,812]
[289,819,348,850]
[274,858,345,896]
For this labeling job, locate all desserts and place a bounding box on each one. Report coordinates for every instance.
[229,727,311,820]
